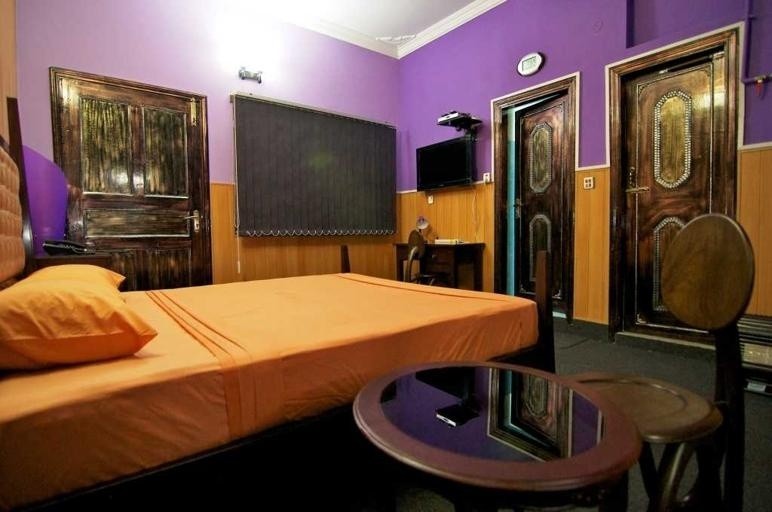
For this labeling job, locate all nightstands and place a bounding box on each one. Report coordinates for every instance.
[27,254,114,281]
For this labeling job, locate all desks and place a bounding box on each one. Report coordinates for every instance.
[393,242,486,292]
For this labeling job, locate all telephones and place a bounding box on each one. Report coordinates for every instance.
[42,238,99,257]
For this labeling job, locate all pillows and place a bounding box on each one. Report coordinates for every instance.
[23,265,126,294]
[0,264,158,369]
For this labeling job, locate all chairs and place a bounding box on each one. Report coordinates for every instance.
[403,230,449,286]
[566,211,757,511]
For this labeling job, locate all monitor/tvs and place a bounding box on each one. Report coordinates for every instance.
[415,367,476,398]
[416,134,477,191]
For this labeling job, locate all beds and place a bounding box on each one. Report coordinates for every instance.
[0,145,557,512]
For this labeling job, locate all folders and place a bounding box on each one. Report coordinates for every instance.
[434,240,469,248]
